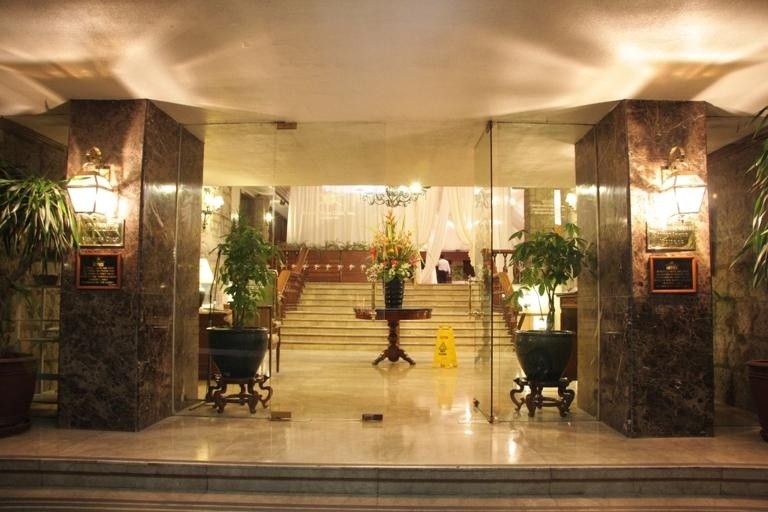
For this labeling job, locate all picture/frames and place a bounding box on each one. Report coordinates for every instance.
[650,255,697,293]
[78,219,125,247]
[76,253,121,289]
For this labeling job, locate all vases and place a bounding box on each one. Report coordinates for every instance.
[384,281,405,308]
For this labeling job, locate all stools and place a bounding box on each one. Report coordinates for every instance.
[206,374,273,413]
[510,377,577,417]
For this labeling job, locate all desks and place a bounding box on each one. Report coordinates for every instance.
[353,309,432,365]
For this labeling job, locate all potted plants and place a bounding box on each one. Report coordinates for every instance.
[0,173,102,439]
[206,202,285,377]
[33,240,58,286]
[729,105,768,443]
[503,224,598,381]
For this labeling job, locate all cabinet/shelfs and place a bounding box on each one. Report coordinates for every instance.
[199,308,232,380]
[553,293,577,381]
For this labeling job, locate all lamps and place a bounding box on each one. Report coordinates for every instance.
[65,147,114,220]
[659,147,707,219]
[199,258,214,308]
[201,186,224,229]
[511,271,537,311]
[360,186,431,207]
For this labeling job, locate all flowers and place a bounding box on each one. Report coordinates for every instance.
[365,206,421,283]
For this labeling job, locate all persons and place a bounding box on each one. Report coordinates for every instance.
[436,254,451,283]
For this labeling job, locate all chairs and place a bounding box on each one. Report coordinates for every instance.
[232,306,281,377]
